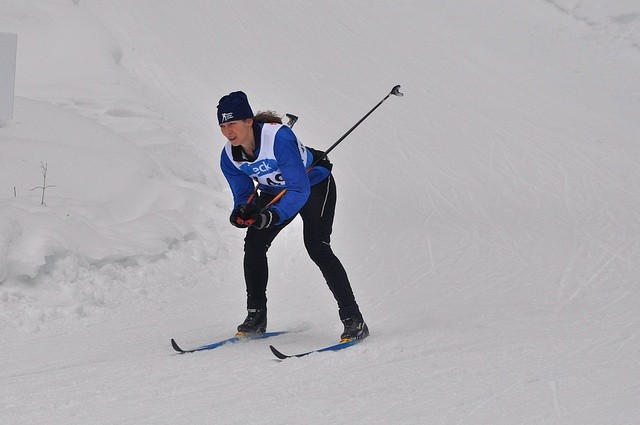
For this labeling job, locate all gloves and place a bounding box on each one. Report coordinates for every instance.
[251,209,279,230]
[230,204,254,228]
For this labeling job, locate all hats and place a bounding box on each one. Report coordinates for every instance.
[216,91,254,124]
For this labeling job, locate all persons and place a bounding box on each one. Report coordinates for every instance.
[217,91,370,341]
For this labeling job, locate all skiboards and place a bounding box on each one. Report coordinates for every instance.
[170,330,362,359]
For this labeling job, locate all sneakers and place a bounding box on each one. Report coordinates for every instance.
[238,308,267,334]
[341,311,368,340]
